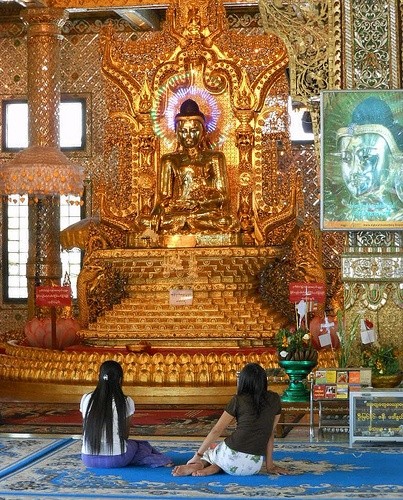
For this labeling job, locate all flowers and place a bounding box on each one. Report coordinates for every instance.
[277,325,313,357]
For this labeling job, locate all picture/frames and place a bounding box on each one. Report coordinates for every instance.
[320,89,403,231]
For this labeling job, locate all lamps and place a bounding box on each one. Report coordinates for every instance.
[0,147,88,320]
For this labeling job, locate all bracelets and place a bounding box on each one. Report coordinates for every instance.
[196,451,202,457]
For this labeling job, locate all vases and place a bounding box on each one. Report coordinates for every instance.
[278,348,318,364]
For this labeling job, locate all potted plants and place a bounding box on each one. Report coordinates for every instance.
[371,349,403,388]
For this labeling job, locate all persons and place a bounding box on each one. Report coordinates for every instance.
[150,99,232,232]
[170,362,289,476]
[79,361,174,468]
[329,98,403,221]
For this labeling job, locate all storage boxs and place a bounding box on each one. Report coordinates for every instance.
[349,388,403,448]
